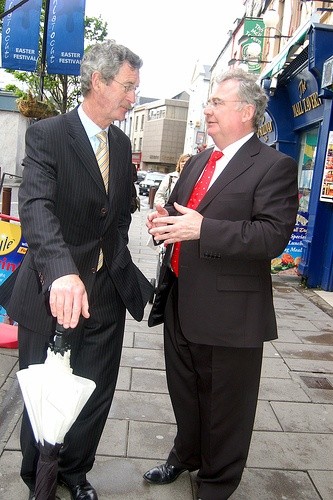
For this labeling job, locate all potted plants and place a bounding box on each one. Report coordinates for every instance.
[16,88,48,119]
[36,97,60,121]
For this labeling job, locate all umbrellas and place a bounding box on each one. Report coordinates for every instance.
[16,320,96,500]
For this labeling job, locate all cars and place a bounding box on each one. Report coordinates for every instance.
[134,170,167,196]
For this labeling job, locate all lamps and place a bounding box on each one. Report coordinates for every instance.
[237,34,293,46]
[227,58,273,67]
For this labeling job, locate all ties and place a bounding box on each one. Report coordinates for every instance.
[171,151,224,278]
[96,131,109,271]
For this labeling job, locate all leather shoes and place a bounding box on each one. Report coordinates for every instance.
[58,475,98,500]
[28,487,36,500]
[143,462,199,484]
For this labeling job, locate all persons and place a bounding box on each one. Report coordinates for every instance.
[148,154,193,280]
[142,66,299,500]
[0,41,156,500]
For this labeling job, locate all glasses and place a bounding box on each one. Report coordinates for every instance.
[202,99,240,109]
[109,77,136,93]
